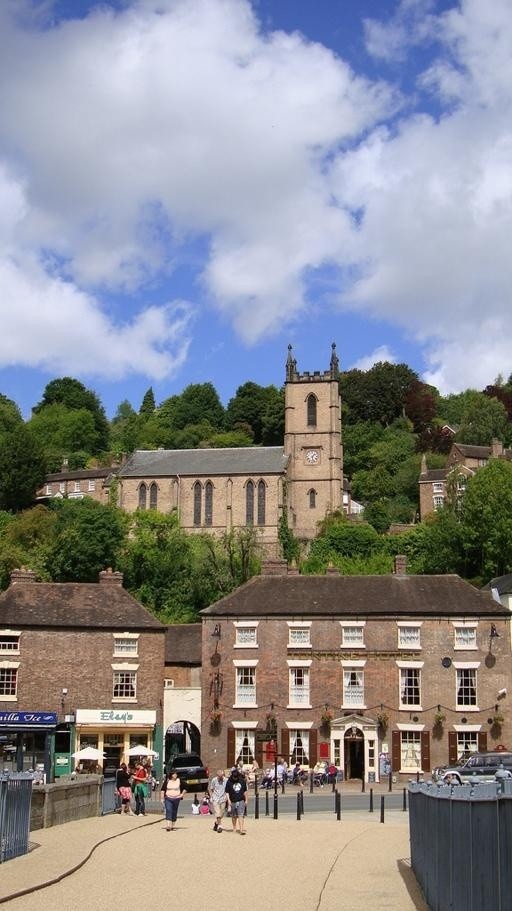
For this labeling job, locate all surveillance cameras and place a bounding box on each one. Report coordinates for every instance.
[63,688,68,695]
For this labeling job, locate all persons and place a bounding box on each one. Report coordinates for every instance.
[231,758,337,791]
[199,802,210,816]
[191,799,200,816]
[225,768,248,835]
[71,765,105,783]
[31,768,45,787]
[160,769,189,832]
[116,759,159,817]
[210,770,228,834]
[202,792,211,813]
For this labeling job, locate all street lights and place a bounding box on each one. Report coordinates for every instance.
[268,739,279,817]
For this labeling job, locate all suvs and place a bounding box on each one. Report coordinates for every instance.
[431,749,512,789]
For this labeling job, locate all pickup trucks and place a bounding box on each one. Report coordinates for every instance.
[164,752,209,791]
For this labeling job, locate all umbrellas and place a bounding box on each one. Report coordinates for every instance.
[123,745,159,761]
[74,750,108,763]
[70,746,107,759]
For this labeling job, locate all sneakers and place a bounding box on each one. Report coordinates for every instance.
[167,827,176,831]
[121,811,144,817]
[214,822,246,834]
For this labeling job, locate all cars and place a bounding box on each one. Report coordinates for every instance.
[0,735,24,763]
